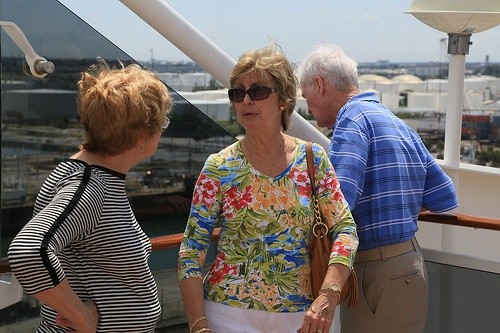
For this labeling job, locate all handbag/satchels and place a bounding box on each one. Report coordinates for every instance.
[306,141,360,309]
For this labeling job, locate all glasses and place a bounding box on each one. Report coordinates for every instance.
[228,85,278,102]
[162,117,170,129]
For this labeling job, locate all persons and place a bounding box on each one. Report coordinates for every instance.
[7,63,171,333]
[300,46,459,333]
[177,48,360,333]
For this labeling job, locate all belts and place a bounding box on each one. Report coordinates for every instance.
[354,237,419,263]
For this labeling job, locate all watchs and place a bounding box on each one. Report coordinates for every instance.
[319,282,341,304]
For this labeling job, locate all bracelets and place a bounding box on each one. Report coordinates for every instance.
[195,328,214,333]
[190,317,208,333]
